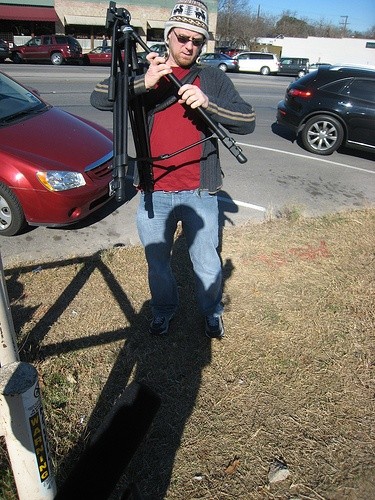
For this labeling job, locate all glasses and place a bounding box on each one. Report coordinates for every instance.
[172,28,204,46]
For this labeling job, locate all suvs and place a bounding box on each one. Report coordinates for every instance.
[276,57,310,78]
[8,34,82,65]
[275,64,375,156]
[232,52,280,76]
[137,43,169,65]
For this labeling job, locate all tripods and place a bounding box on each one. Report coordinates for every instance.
[102,1,244,201]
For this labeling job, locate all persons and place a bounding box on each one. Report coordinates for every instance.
[90,0,255,337]
[30,33,36,39]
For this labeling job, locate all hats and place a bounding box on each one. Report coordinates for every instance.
[164,0,210,47]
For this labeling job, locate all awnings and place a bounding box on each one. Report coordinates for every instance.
[148,21,166,29]
[0,4,58,22]
[65,15,142,27]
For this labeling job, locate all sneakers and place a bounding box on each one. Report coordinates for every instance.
[149,312,174,335]
[204,313,224,339]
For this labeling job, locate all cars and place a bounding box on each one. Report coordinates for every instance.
[196,52,238,73]
[81,46,124,67]
[0,38,16,62]
[0,72,116,238]
[298,62,332,78]
